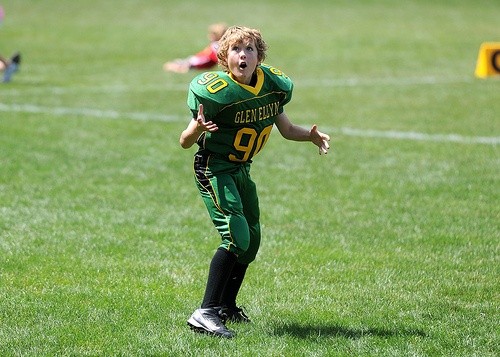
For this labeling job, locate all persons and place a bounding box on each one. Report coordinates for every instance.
[180,25,330,336]
[164,22,230,74]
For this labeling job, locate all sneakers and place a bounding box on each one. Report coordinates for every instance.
[187,306,237,338]
[218,305,251,322]
